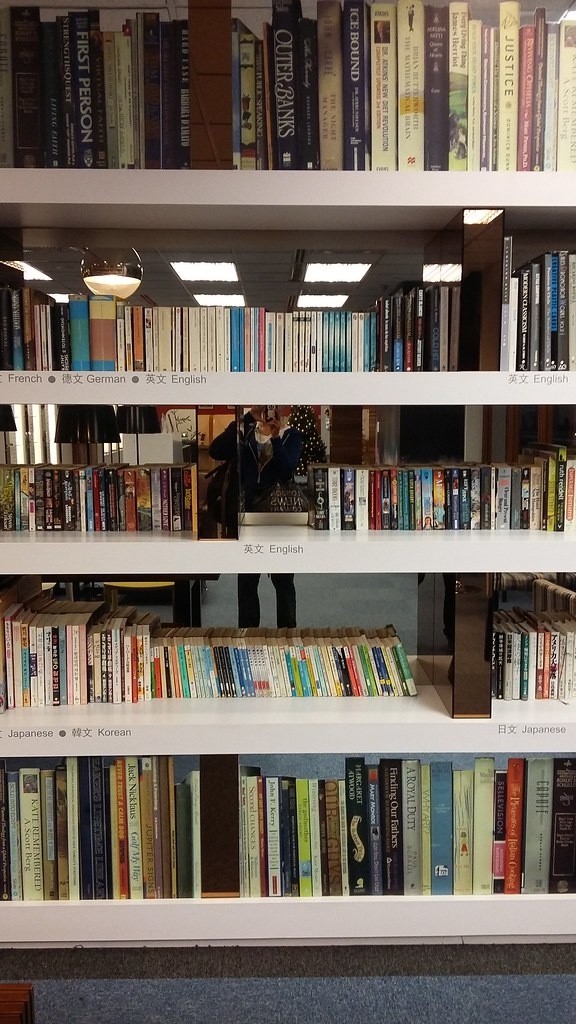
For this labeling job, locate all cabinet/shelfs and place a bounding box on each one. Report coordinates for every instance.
[0,0,576,948]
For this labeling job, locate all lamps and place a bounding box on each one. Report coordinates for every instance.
[54,404,122,444]
[116,406,163,434]
[80,248,143,299]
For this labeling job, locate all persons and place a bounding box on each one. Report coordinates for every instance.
[374,21,390,43]
[208,405,329,628]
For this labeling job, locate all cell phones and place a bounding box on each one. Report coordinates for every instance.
[265,406,278,422]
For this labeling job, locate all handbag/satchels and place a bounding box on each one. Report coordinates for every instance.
[205,461,237,524]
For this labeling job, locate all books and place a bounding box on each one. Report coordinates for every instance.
[492,619,576,701]
[0,753,576,901]
[22,591,396,637]
[493,605,571,625]
[499,237,576,371]
[0,463,197,532]
[0,0,575,171]
[1,604,418,709]
[1,286,460,372]
[311,438,575,532]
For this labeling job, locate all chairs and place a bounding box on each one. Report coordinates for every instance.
[103,582,175,628]
